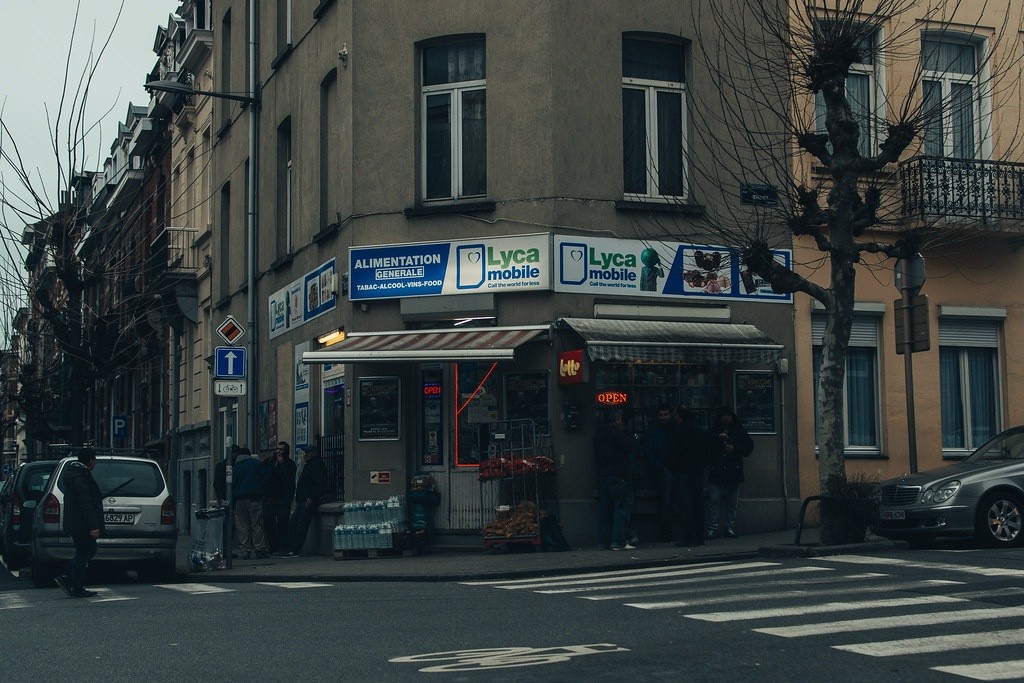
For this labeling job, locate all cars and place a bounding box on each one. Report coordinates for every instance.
[0,458,62,572]
[864,424,1024,549]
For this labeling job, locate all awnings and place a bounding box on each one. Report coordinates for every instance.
[557,316,786,365]
[301,323,554,365]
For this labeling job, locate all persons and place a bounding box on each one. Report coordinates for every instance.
[213,442,297,554]
[591,408,643,551]
[54,449,106,598]
[640,403,754,548]
[287,445,325,556]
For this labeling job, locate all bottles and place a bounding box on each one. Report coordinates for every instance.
[637,366,705,385]
[334,495,403,548]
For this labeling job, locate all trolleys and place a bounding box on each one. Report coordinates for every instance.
[476,417,554,553]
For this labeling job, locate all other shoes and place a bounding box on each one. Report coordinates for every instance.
[242,550,251,560]
[723,528,737,537]
[53,576,72,597]
[597,544,610,550]
[675,539,704,547]
[704,527,718,540]
[662,532,672,542]
[223,550,238,558]
[68,584,98,598]
[612,542,636,550]
[268,544,300,558]
[256,550,266,558]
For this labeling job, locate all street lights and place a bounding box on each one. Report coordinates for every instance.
[138,1,262,450]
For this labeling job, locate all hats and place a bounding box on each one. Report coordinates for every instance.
[301,444,318,451]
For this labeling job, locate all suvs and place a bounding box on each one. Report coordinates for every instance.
[21,444,181,586]
